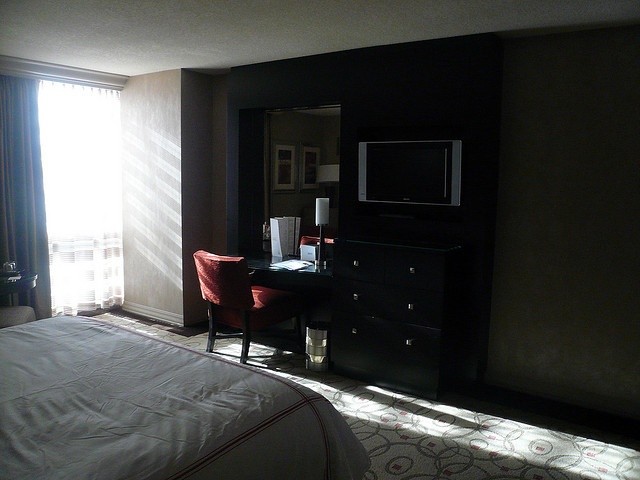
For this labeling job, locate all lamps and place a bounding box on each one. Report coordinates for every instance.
[316,198,329,262]
[318,165,339,207]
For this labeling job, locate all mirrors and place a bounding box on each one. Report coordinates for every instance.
[264,104,341,226]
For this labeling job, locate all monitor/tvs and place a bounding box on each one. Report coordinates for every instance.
[357,137,463,206]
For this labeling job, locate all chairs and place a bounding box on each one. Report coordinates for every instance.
[193,249,305,364]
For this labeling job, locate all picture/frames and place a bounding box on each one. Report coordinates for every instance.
[271,144,297,193]
[299,146,320,189]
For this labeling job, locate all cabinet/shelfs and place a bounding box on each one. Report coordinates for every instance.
[330,236,495,402]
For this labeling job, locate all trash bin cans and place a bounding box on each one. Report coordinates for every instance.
[305,322,330,371]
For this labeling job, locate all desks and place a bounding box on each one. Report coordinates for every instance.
[303,208,337,226]
[245,246,332,276]
[1,270,37,307]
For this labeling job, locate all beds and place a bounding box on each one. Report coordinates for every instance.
[0,315,370,479]
